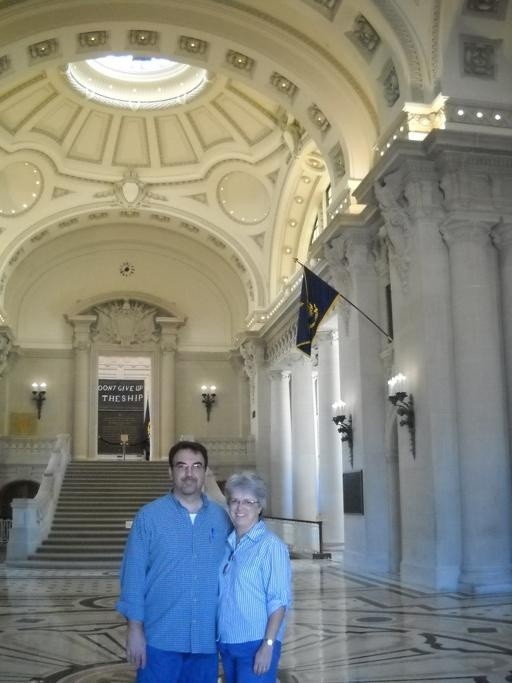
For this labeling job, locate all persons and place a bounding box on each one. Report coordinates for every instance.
[214,469,294,682]
[115,440,233,682]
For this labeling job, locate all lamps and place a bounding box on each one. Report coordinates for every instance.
[330,398,355,470]
[386,372,418,462]
[32,382,47,420]
[200,383,217,422]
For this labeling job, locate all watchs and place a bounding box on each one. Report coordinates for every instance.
[263,636,275,646]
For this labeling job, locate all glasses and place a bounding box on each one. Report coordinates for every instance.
[172,463,205,471]
[229,497,260,507]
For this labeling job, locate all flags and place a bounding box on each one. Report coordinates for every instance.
[297,266,339,358]
